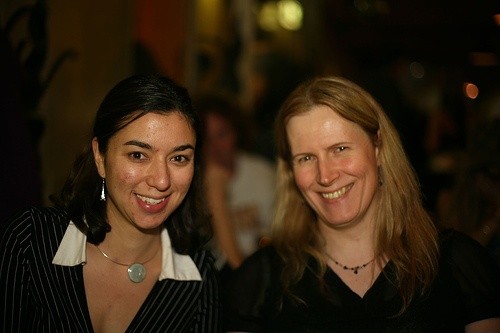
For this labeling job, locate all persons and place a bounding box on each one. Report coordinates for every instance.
[225,75,500,333]
[201,29,285,270]
[0,77,224,333]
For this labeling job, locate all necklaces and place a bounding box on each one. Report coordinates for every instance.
[324,248,385,273]
[96,231,161,283]
